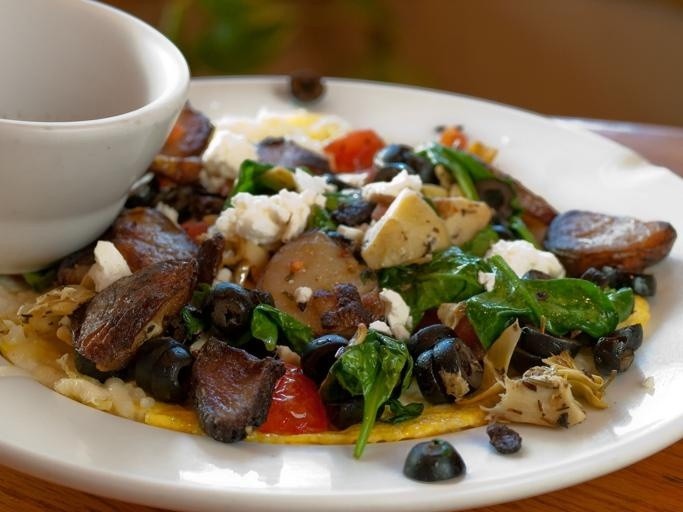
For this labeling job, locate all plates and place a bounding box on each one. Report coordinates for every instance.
[0,73,682,510]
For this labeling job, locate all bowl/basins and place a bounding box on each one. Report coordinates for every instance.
[1,1,190,277]
